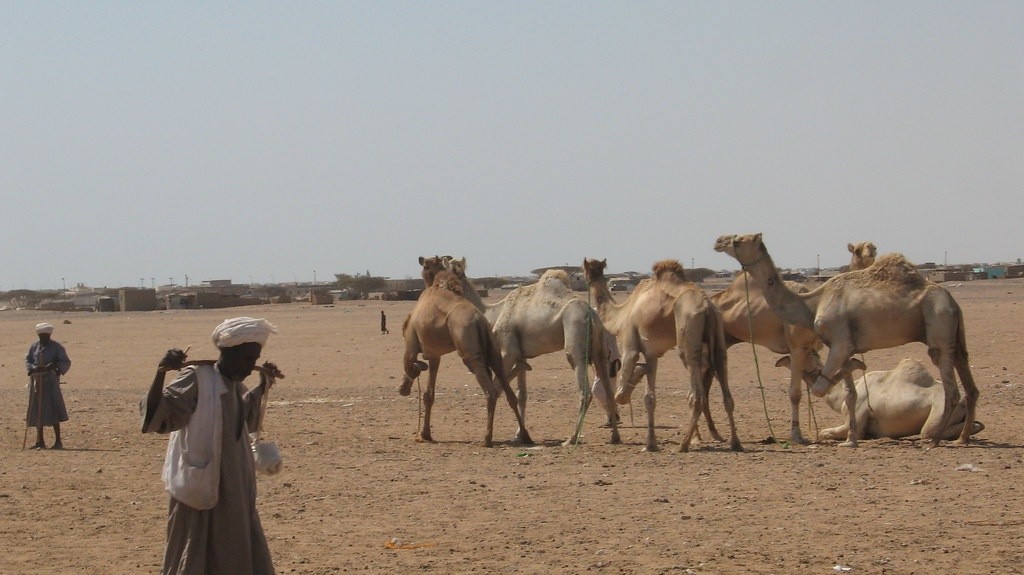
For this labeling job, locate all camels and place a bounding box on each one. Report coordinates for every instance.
[400,232,986,450]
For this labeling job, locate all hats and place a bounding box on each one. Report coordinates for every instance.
[36,323,53,335]
[212,316,278,348]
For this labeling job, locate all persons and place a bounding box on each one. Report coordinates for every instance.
[381,311,390,334]
[25,322,73,450]
[138,314,282,575]
